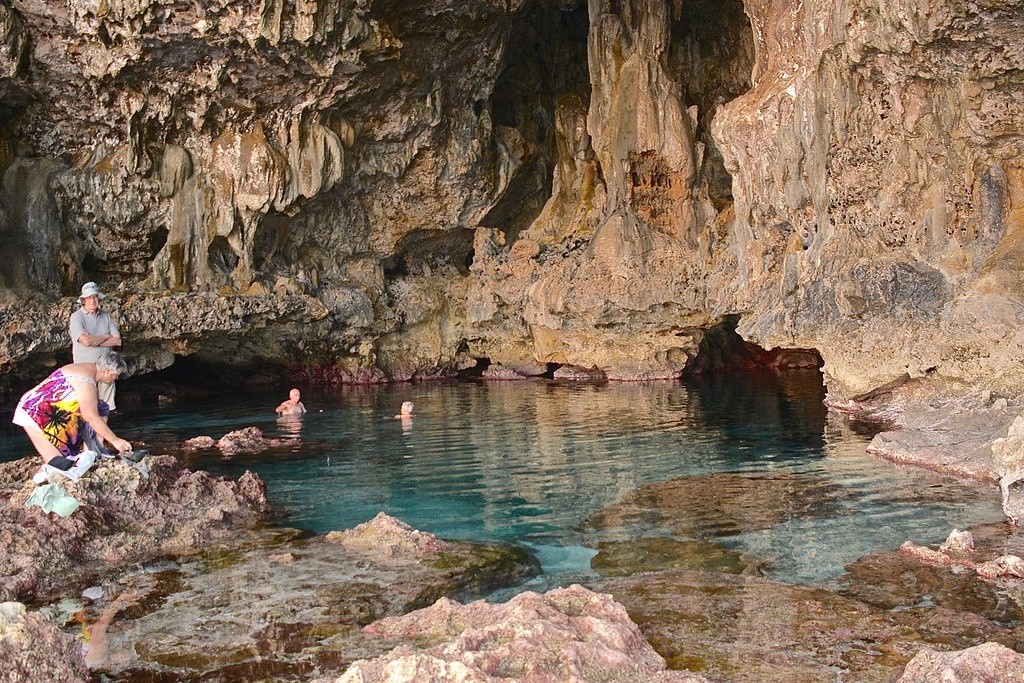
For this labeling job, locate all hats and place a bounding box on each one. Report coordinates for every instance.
[76,282,106,303]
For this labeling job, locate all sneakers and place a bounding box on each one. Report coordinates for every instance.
[97,444,115,458]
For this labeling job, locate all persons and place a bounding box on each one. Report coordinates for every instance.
[69,280,122,452]
[394,402,414,420]
[275,388,307,416]
[11,350,132,466]
